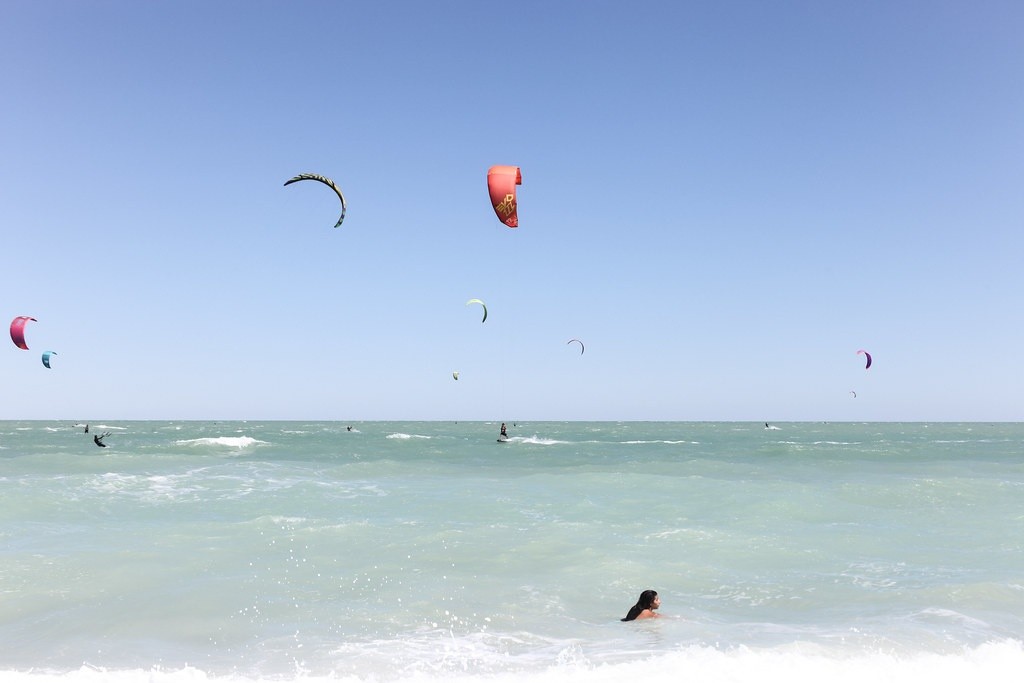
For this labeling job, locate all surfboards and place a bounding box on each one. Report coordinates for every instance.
[497,439,506,442]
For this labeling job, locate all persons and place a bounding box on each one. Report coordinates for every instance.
[85,425,89,433]
[347,426,352,431]
[501,423,508,439]
[622,590,666,621]
[94,435,105,447]
